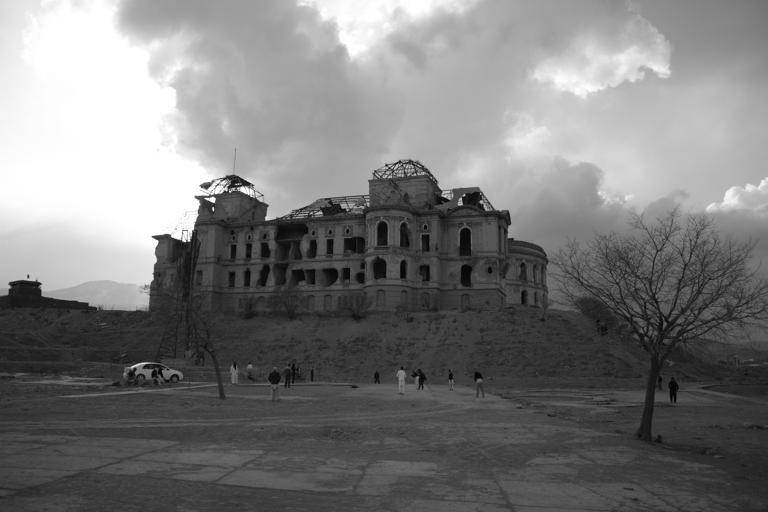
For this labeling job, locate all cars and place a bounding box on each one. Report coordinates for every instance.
[123,362,183,384]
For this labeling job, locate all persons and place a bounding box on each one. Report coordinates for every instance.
[230,360,238,384]
[658,373,663,391]
[474,370,486,399]
[396,366,407,394]
[374,369,381,385]
[158,367,164,385]
[151,367,158,386]
[280,362,293,389]
[269,367,281,402]
[669,376,680,403]
[310,368,314,382]
[448,369,455,391]
[410,372,419,388]
[415,369,426,390]
[290,363,296,385]
[247,362,253,379]
